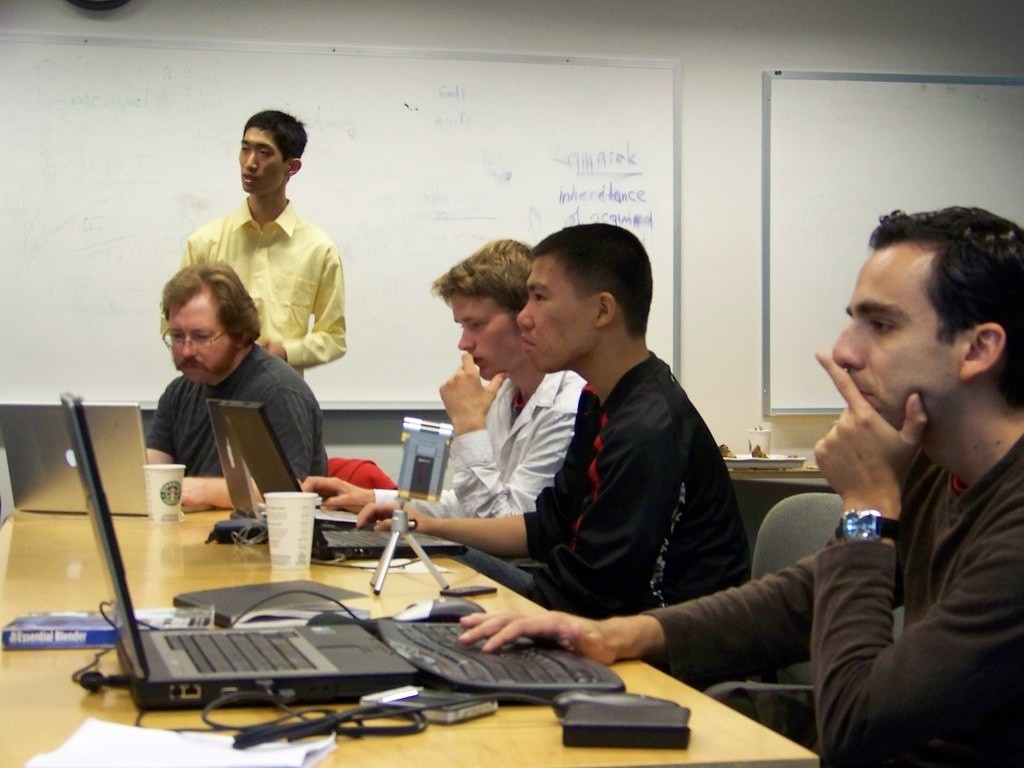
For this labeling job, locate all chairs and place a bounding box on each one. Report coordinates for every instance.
[750,493,904,682]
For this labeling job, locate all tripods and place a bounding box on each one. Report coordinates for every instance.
[369,501,451,595]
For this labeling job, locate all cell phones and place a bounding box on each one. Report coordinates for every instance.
[440,586,496,597]
[362,685,498,725]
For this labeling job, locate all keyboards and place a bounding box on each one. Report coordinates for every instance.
[377,619,625,704]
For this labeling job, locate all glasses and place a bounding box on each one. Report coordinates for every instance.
[162,328,224,349]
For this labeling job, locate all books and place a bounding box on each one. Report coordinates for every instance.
[2,605,216,650]
[173,580,371,628]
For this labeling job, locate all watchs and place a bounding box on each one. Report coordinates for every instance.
[835,509,901,544]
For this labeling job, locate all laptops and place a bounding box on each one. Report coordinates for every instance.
[204,398,468,561]
[59,393,418,711]
[0,404,216,516]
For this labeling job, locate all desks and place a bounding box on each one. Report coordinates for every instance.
[1,514,820,768]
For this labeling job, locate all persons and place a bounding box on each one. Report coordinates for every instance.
[299,239,585,607]
[145,263,330,508]
[461,207,1024,768]
[356,221,782,692]
[183,112,348,380]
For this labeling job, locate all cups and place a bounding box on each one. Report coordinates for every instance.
[143,464,186,523]
[263,492,318,570]
[748,429,772,459]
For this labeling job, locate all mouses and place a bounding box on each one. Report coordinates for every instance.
[392,597,485,622]
[552,688,679,720]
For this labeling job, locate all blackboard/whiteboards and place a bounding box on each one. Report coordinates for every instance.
[0,31,682,411]
[762,70,1024,419]
